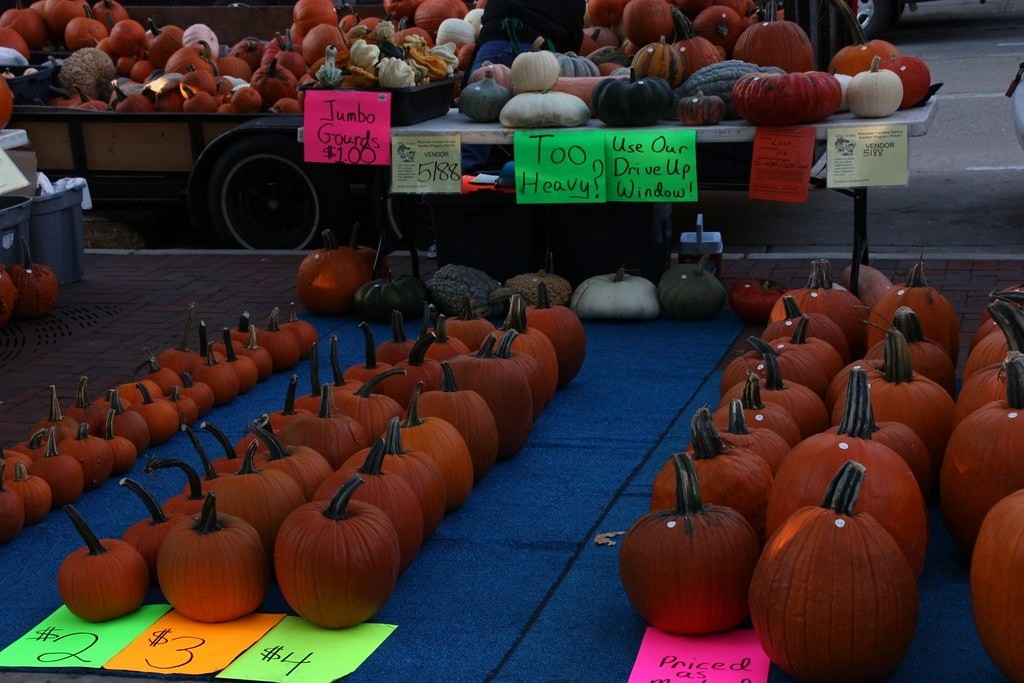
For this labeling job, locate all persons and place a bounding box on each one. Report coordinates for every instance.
[460,0,586,176]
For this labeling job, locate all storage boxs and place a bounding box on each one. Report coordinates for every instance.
[26,181,90,285]
[0,192,33,277]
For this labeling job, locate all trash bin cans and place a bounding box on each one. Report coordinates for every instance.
[30,180,85,286]
[0,194,35,270]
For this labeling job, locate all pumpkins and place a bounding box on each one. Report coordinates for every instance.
[0,299,318,547]
[56,281,586,630]
[460,0,930,128]
[618,238,1024,683]
[296,223,795,319]
[0,0,488,122]
[0,235,58,325]
